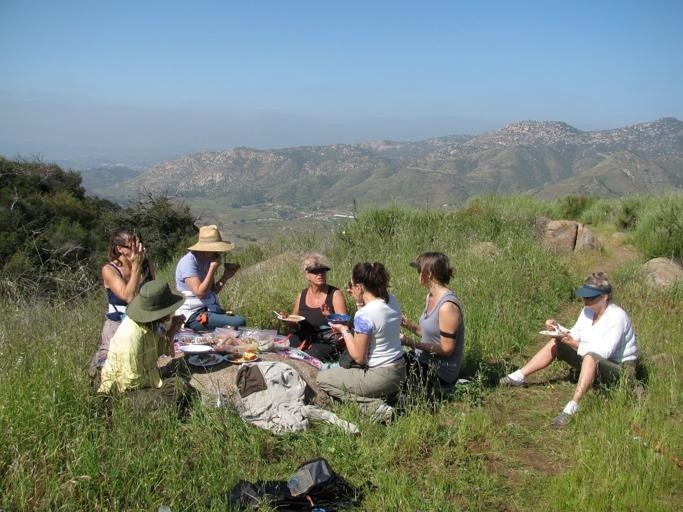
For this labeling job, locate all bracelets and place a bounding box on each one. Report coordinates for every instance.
[341,329,354,336]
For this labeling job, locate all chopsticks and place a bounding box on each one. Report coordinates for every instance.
[539,331,567,338]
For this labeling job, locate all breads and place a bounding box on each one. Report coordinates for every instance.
[244,351,256,361]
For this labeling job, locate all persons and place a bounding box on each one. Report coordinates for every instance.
[498,272,639,425]
[399,251,465,389]
[174,224,247,330]
[285,254,348,360]
[314,261,409,425]
[92,228,167,368]
[97,279,190,418]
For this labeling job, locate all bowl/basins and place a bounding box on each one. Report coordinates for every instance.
[224,263,237,273]
[327,314,351,324]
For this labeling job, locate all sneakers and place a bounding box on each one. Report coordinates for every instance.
[550,410,583,427]
[489,373,529,388]
[370,392,407,424]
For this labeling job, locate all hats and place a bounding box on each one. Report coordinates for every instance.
[574,283,611,298]
[186,224,235,252]
[408,262,421,272]
[126,280,185,324]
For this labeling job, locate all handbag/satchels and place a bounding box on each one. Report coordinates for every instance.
[229,458,363,512]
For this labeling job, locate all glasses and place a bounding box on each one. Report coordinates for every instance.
[117,242,134,250]
[306,266,330,274]
[348,281,360,290]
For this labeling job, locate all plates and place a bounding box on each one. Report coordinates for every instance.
[180,345,258,367]
[277,314,306,323]
